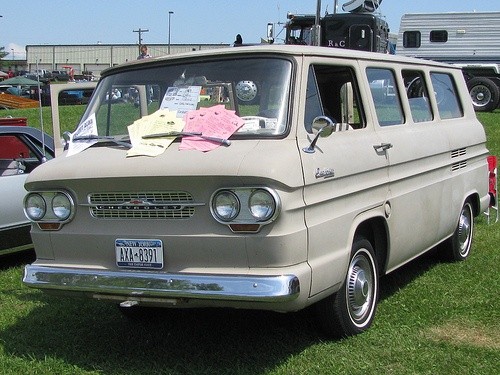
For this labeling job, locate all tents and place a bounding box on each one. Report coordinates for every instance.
[0,74,43,96]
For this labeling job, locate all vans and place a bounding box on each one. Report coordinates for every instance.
[21,43,490,335]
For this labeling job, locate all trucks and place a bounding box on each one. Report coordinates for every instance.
[260,0,500,112]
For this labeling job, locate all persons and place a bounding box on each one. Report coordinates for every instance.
[135,46,152,108]
[105,85,137,104]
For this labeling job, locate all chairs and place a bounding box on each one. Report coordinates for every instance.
[0,159,26,176]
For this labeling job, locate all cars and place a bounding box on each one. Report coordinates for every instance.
[25,69,49,77]
[0,124,69,261]
